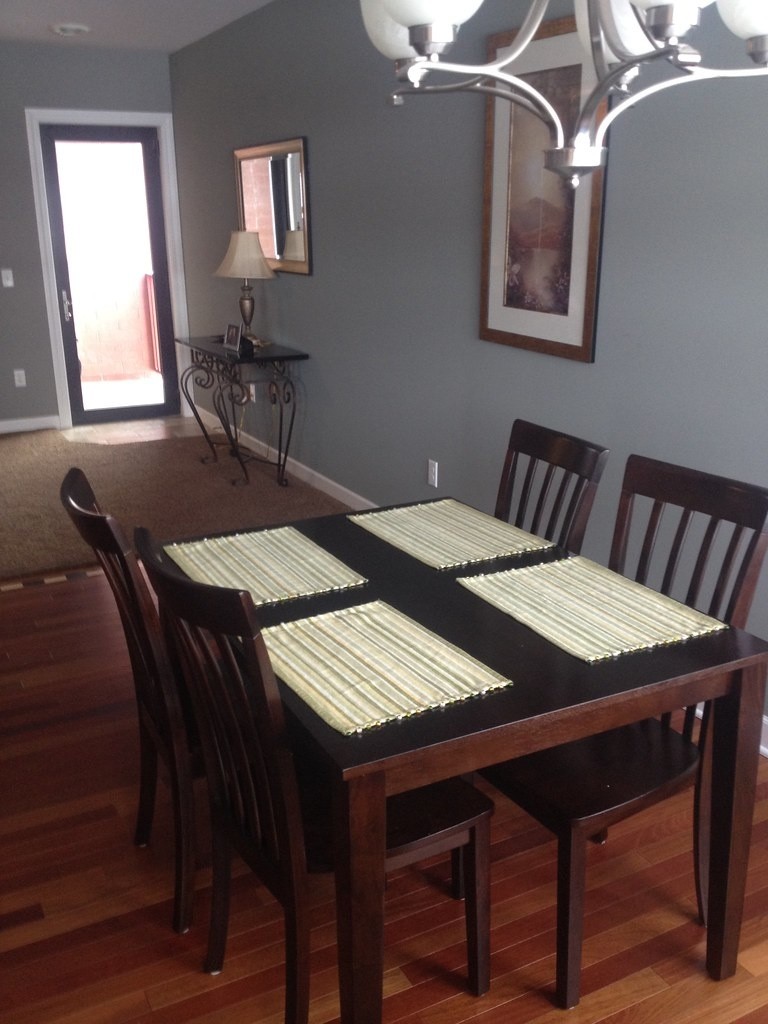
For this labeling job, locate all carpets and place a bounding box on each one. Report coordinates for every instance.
[1,426,361,594]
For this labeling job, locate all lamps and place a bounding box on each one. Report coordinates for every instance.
[210,231,279,345]
[280,222,306,262]
[358,1,768,190]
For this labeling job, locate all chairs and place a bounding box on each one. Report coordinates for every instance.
[129,520,502,1024]
[444,448,768,1014]
[58,464,229,938]
[489,417,612,556]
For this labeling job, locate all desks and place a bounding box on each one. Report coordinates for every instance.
[134,494,768,1024]
[174,332,311,489]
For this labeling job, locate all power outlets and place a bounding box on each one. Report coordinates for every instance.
[428,458,439,488]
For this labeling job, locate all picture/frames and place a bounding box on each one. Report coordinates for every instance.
[222,320,243,352]
[475,12,614,365]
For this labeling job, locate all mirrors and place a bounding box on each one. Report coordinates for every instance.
[231,137,312,278]
